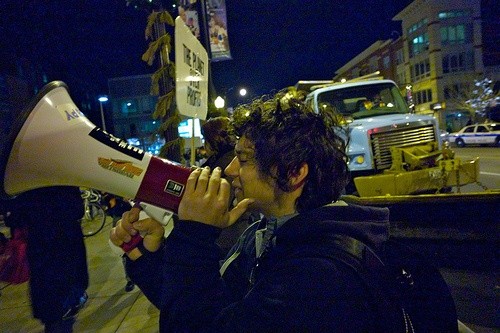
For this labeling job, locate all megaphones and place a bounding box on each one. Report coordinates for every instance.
[0,80,211,253]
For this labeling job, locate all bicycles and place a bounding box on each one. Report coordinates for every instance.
[80,189,106,237]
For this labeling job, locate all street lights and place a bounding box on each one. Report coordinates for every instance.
[222,86,247,111]
[98,94,109,130]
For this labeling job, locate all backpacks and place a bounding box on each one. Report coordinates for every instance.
[268,230,458,333]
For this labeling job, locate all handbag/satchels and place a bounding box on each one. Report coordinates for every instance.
[0,237,29,284]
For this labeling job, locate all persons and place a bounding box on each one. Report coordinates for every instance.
[182,145,208,169]
[110,86,404,333]
[92,189,136,292]
[5,184,89,333]
[176,4,228,45]
[199,116,262,258]
[360,90,387,111]
[465,116,475,132]
[481,118,500,132]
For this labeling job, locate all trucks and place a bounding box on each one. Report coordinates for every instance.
[295,71,481,197]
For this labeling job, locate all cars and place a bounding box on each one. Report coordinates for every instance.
[448,122,500,146]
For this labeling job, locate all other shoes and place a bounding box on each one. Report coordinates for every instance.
[126,281,135,291]
